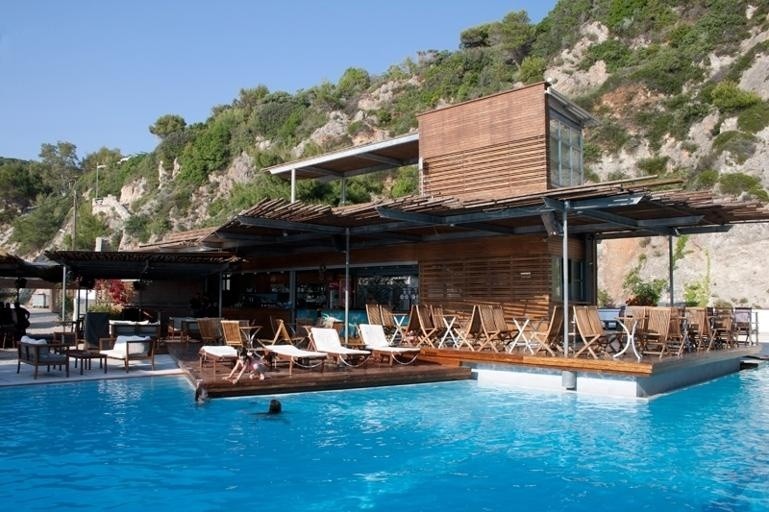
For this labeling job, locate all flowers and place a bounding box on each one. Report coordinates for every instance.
[95,279,135,312]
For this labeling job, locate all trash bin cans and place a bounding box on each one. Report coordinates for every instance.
[85,312,109,348]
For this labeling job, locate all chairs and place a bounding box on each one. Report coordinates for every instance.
[362,300,752,362]
[195,309,424,385]
[16,313,193,381]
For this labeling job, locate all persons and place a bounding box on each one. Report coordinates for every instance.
[193,386,209,405]
[267,399,282,416]
[222,347,269,384]
[0,300,30,348]
